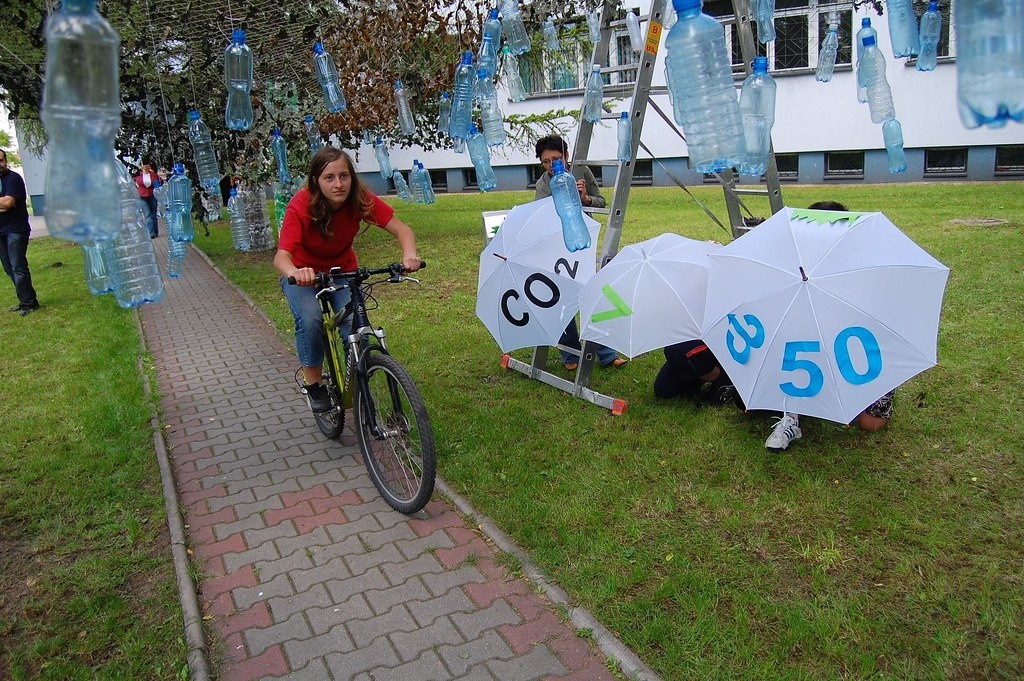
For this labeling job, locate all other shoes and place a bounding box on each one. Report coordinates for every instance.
[11,303,23,311]
[151,235,158,239]
[19,303,40,316]
[564,361,578,370]
[599,358,628,369]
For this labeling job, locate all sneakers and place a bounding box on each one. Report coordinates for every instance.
[302,382,330,413]
[764,394,803,452]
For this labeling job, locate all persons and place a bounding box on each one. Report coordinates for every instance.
[534,134,628,371]
[764,201,895,452]
[0,148,40,317]
[132,165,164,239]
[273,147,422,414]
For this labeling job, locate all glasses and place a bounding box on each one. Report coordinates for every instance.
[540,154,563,167]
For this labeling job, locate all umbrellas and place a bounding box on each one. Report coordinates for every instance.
[475,191,602,355]
[578,233,725,361]
[700,205,952,428]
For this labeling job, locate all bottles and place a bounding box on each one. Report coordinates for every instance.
[224,28,255,131]
[663,0,777,177]
[438,0,531,191]
[548,158,591,253]
[586,9,602,43]
[543,20,558,51]
[313,43,346,113]
[617,111,632,163]
[394,80,416,136]
[953,0,1024,130]
[856,17,907,175]
[373,133,436,206]
[40,0,223,308]
[815,24,838,83]
[272,115,323,185]
[885,0,942,72]
[226,188,250,251]
[625,7,644,52]
[584,64,604,123]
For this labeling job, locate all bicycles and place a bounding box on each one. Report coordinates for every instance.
[288,259,435,514]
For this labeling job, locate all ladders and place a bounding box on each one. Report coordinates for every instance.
[500,0,784,417]
